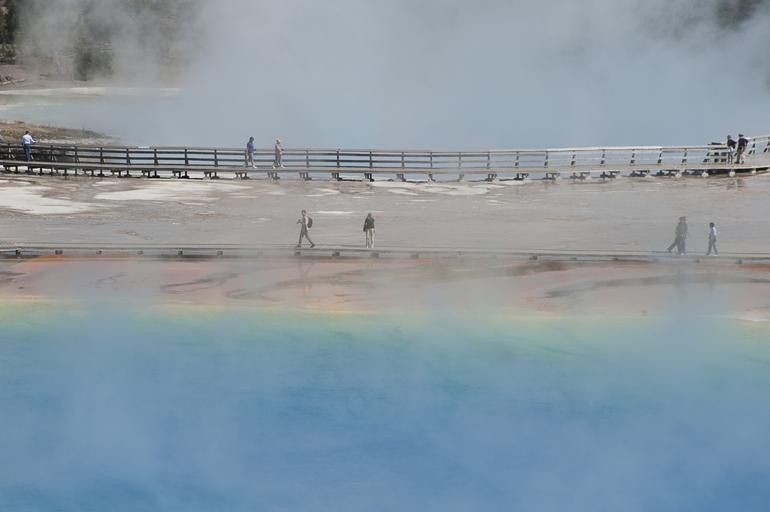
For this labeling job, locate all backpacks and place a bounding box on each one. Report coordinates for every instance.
[307,217,313,228]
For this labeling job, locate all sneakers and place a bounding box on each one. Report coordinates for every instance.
[243,164,286,170]
[295,243,316,248]
[366,245,374,248]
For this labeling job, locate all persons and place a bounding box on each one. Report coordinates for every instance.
[726,134,737,165]
[734,133,748,164]
[706,222,719,255]
[247,137,258,169]
[364,213,375,248]
[272,139,285,169]
[295,209,315,247]
[21,130,36,161]
[668,216,689,254]
